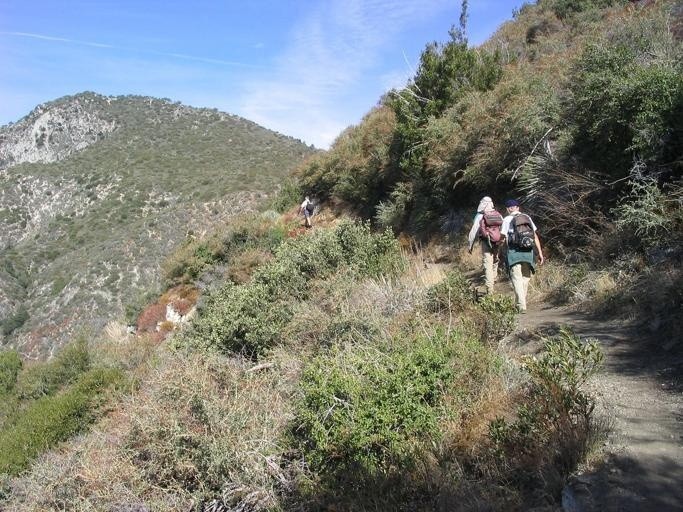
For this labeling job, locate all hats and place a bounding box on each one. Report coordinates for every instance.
[477,196,494,211]
[504,199,520,207]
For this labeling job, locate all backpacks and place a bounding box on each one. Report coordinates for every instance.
[306,202,314,211]
[507,213,536,252]
[477,210,505,251]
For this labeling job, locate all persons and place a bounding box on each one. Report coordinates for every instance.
[500,200,543,314]
[300,196,312,228]
[468,196,503,296]
